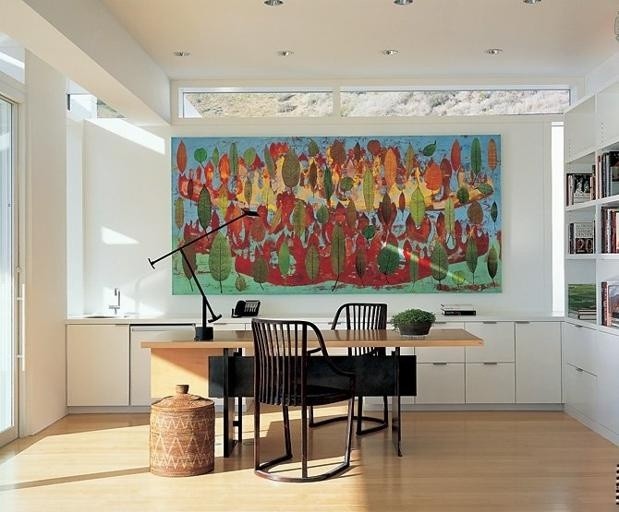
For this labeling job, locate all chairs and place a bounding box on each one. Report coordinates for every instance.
[250,302,388,483]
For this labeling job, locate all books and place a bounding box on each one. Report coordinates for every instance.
[442,308,478,317]
[566,148,619,330]
[441,303,480,310]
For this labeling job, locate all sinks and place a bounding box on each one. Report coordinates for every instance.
[84,314,117,319]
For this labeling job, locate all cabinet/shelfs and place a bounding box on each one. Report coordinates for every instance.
[563,77,619,444]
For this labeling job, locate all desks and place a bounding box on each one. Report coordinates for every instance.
[139,329,484,456]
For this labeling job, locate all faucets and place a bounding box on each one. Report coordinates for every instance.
[107,286,121,313]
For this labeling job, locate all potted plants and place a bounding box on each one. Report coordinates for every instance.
[393,308,435,336]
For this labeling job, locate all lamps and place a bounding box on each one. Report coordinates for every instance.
[148,209,260,322]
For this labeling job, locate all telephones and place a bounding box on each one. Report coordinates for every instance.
[234,299,261,316]
